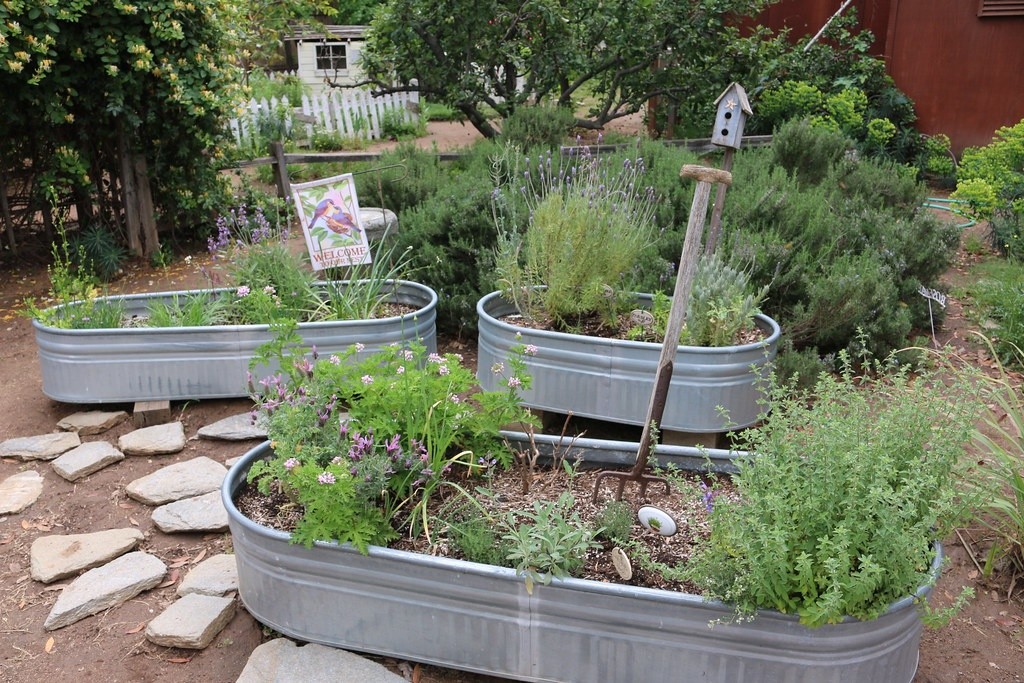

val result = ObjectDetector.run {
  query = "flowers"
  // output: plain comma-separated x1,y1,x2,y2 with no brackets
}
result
4,197,426,327
232,313,1011,635
488,131,786,346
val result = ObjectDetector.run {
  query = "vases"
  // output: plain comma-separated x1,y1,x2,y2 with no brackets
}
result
219,432,945,683
473,284,780,434
31,278,437,405
